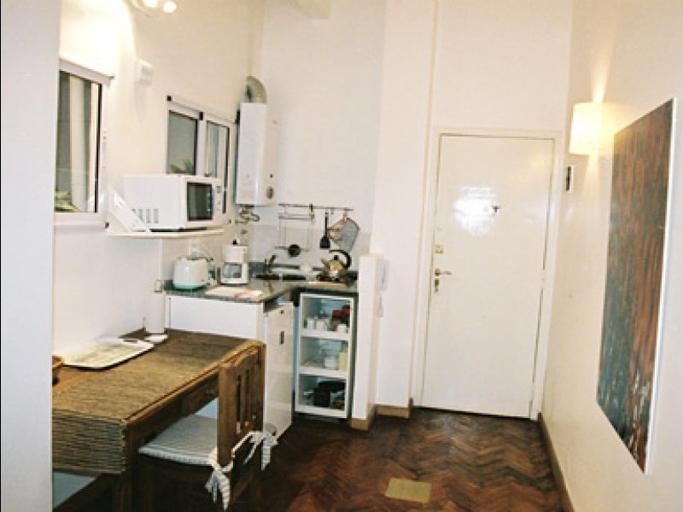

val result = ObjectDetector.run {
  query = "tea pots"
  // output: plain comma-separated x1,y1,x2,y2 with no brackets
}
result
320,248,351,277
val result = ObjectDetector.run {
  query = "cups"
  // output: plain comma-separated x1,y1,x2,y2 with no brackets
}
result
314,388,330,406
316,320,328,331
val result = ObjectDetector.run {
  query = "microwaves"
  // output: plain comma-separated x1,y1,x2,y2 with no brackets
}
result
124,174,223,231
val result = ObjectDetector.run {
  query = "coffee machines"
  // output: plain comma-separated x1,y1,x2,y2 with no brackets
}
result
220,244,248,284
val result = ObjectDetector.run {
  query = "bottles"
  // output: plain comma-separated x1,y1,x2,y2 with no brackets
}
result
208,257,217,285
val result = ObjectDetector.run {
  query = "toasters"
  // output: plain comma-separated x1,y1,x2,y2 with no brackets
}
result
174,255,208,291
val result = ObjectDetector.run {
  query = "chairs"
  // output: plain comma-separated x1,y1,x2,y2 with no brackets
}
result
134,340,262,512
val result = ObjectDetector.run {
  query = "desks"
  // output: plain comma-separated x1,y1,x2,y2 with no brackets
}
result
51,327,264,512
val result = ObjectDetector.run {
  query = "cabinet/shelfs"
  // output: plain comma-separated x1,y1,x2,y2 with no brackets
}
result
295,292,356,423
